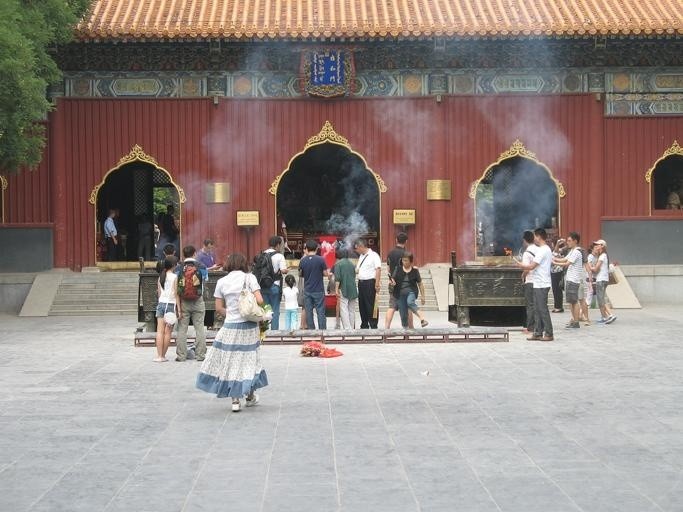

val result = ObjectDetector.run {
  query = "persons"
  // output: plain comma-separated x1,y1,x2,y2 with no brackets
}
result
196,253,268,411
154,205,221,361
519,227,617,341
105,210,119,261
263,232,429,329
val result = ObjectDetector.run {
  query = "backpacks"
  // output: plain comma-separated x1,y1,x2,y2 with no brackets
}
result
250,250,282,289
175,260,204,301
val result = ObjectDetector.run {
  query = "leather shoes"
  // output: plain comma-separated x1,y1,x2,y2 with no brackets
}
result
526,334,543,341
539,335,554,342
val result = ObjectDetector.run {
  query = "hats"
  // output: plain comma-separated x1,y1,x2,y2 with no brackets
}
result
592,239,606,247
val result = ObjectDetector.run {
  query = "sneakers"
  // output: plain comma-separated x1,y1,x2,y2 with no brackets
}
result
551,308,590,328
605,315,618,325
420,318,428,328
244,393,259,407
596,318,607,323
151,355,204,366
230,400,241,412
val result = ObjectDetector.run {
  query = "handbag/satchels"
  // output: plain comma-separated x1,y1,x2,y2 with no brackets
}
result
608,270,620,284
236,273,266,322
163,312,178,326
